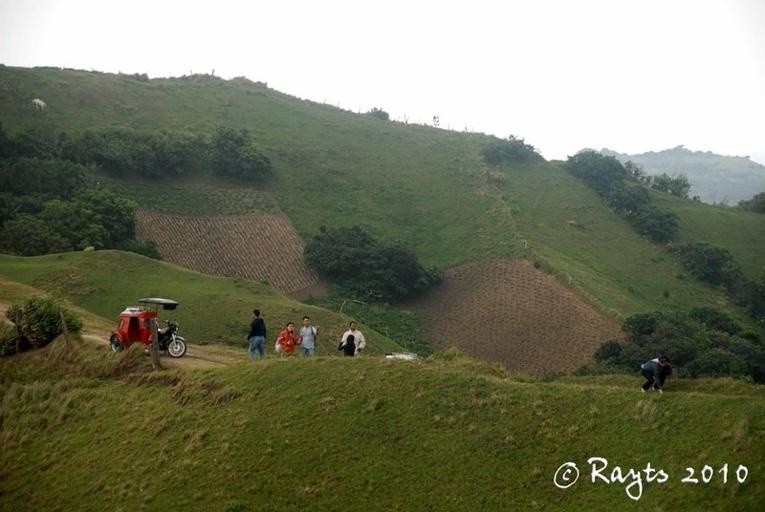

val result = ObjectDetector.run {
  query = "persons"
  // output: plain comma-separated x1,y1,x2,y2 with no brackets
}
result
642,355,672,390
246,308,268,360
300,315,320,356
338,320,366,357
638,357,669,395
278,321,302,358
338,333,356,357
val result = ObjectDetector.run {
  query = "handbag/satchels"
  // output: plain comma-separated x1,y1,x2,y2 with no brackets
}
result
274,337,284,353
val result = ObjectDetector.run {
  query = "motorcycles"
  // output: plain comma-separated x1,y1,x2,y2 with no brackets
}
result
110,297,186,358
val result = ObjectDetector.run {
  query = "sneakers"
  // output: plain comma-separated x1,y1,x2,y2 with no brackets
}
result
640,385,656,393
